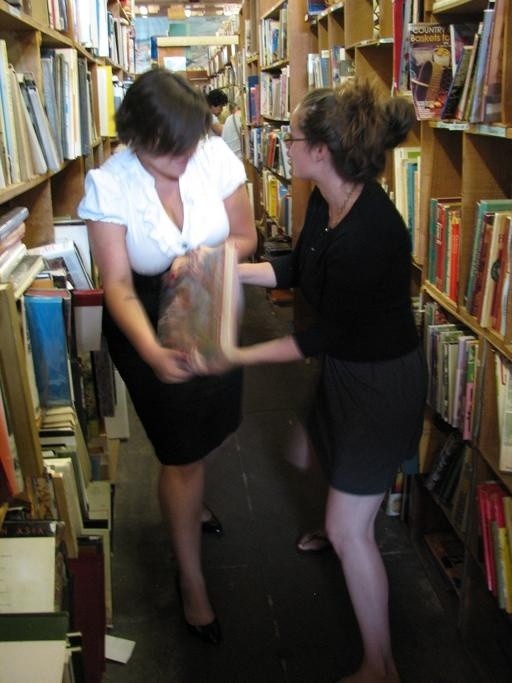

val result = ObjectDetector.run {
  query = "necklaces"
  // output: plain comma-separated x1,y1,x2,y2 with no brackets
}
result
329,178,360,225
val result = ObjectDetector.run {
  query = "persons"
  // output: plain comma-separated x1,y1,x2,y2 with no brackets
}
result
233,78,429,682
77,66,257,650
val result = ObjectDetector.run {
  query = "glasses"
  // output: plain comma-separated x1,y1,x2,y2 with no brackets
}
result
283,132,307,146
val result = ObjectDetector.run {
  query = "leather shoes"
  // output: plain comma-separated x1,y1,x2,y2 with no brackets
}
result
199,502,223,537
173,575,220,645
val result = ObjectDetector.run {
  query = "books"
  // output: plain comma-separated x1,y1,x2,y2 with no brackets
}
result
157,239,235,370
374,1,512,611
1,0,118,682
118,19,138,104
206,0,356,241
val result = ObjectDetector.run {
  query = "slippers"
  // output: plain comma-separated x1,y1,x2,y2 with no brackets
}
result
298,530,330,555
